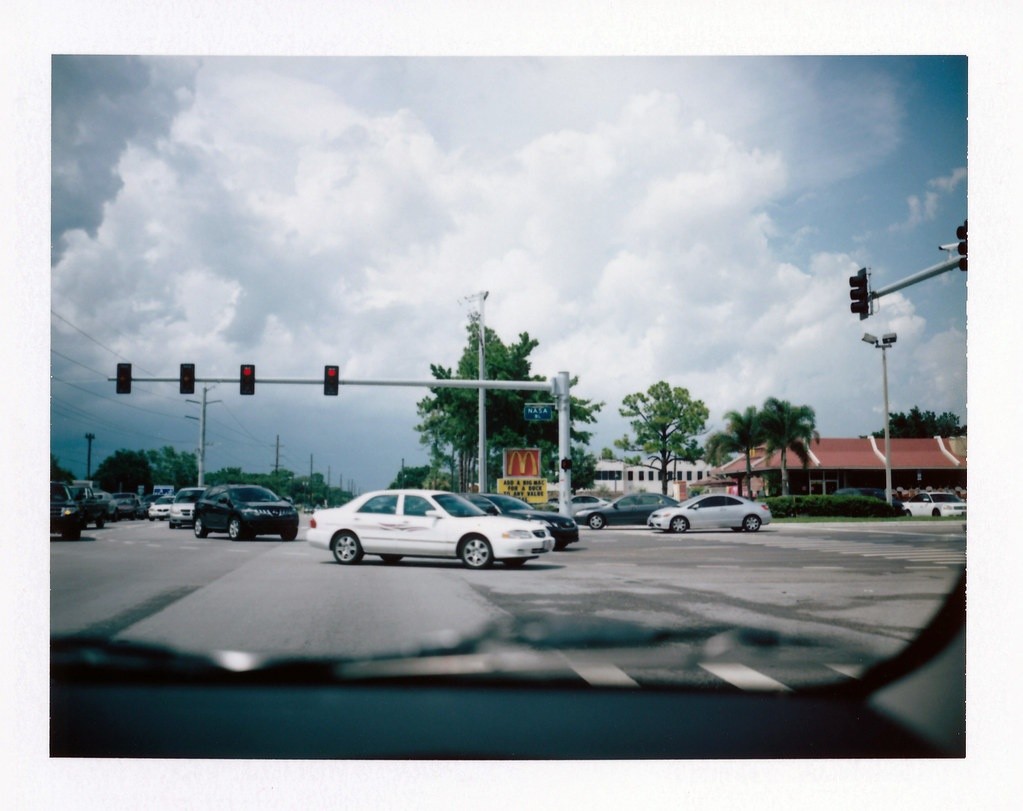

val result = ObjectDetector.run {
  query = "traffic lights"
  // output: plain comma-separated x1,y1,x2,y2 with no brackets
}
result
562,459,574,470
180,364,195,394
956,218,968,271
323,365,339,395
849,268,870,320
116,363,132,394
240,364,255,395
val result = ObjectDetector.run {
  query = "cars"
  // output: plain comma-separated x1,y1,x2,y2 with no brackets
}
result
646,493,774,535
170,487,207,528
148,494,175,521
552,496,611,512
113,494,163,520
418,494,579,551
834,488,904,512
903,491,967,517
575,493,680,530
93,492,120,522
307,489,555,569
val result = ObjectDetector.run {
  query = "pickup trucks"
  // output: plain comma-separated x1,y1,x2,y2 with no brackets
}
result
68,484,109,529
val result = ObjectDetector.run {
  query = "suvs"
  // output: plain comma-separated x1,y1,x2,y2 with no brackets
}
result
50,481,83,539
193,482,299,541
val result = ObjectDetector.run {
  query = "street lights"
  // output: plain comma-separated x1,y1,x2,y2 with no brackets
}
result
862,332,897,506
